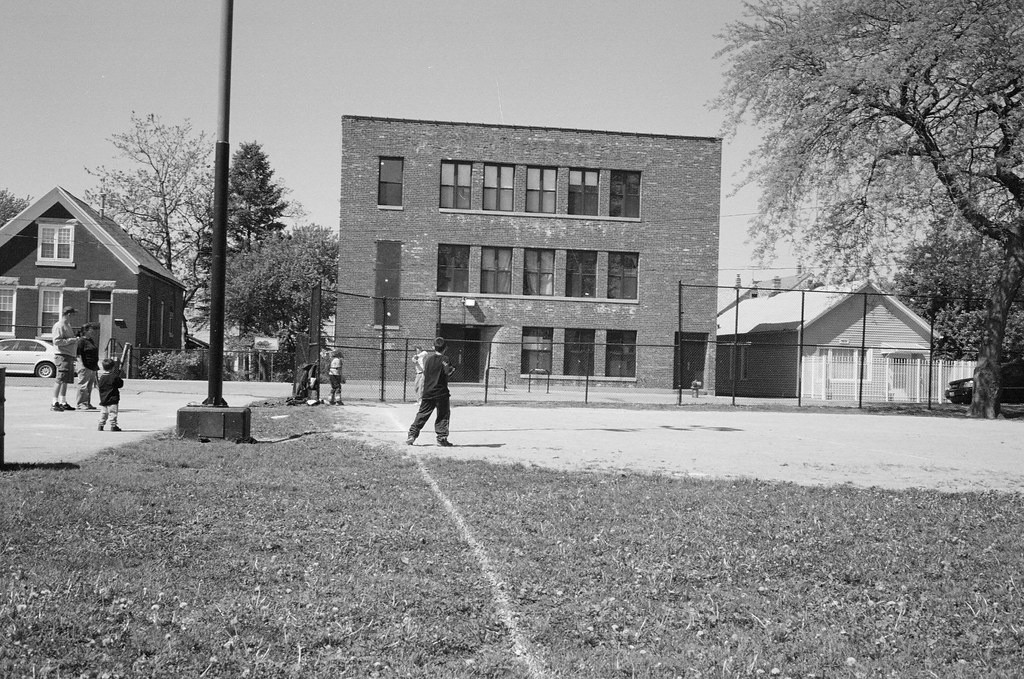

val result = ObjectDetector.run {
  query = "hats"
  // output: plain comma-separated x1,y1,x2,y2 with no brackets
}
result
63,307,78,314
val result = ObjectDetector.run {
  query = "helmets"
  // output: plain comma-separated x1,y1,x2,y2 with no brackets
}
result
332,349,344,357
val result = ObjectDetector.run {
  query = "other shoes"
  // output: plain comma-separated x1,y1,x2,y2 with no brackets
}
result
438,441,453,447
112,426,121,431
414,402,419,406
330,401,344,406
405,437,416,444
98,427,104,431
77,404,97,410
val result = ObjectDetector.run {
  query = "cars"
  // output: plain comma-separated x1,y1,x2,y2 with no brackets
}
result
945,360,1024,404
0,339,62,378
73,356,85,378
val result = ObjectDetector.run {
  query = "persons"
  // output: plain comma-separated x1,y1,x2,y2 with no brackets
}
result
329,349,346,405
406,337,453,446
98,359,124,431
51,306,100,411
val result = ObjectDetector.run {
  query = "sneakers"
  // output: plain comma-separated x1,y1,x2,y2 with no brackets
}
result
50,402,75,411
